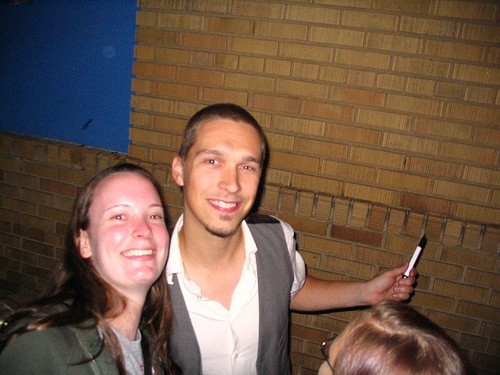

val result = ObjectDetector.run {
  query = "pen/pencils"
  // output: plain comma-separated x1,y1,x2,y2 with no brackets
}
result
402,234,427,279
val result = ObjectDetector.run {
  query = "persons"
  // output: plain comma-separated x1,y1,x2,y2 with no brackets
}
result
317,297,474,375
0,102,418,375
0,154,185,374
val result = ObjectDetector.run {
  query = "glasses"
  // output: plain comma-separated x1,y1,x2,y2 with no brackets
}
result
321,336,339,374
